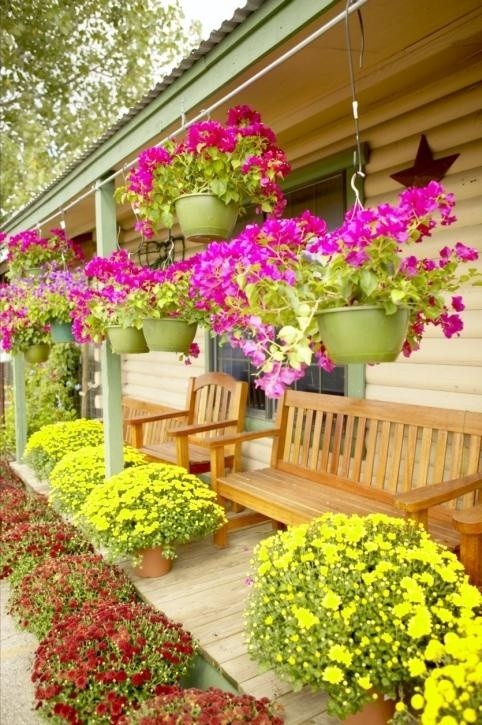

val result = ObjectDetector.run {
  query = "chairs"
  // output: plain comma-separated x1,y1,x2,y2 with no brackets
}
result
124,372,250,515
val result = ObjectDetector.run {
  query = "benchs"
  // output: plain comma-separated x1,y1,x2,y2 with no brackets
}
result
207,389,481,588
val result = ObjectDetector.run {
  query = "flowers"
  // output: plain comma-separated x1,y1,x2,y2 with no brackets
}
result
110,103,288,242
